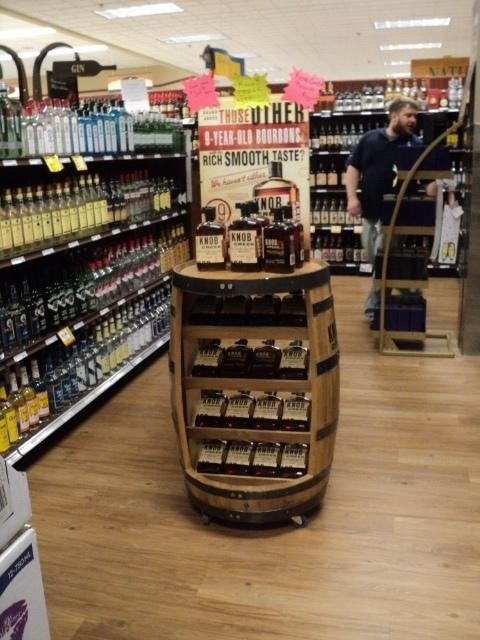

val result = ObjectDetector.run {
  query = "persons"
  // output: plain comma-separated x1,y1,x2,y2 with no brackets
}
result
346,95,438,330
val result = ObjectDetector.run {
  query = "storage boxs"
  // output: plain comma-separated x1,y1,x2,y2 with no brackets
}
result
404,144,450,178
383,191,436,232
377,292,425,350
374,247,430,282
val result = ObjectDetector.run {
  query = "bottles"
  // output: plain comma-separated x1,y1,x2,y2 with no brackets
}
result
247,293,281,325
186,291,221,326
278,441,310,479
250,388,283,430
339,157,349,190
156,221,191,276
309,157,339,191
195,438,229,474
85,299,154,394
252,160,298,222
217,294,249,325
276,340,310,381
0,184,53,260
85,231,158,313
192,205,228,272
457,229,468,267
279,391,313,433
358,169,366,188
278,289,307,328
221,389,256,429
191,338,226,379
151,171,188,215
446,76,466,110
449,158,472,192
1,339,87,456
262,204,306,274
311,231,371,264
0,269,84,351
315,88,334,114
333,87,361,113
53,172,112,248
223,438,257,477
143,90,198,118
218,338,253,378
246,338,282,379
0,79,23,161
146,281,174,339
248,438,285,478
401,235,432,268
365,119,388,130
384,78,430,112
194,388,228,428
77,96,135,157
310,119,365,153
133,111,186,155
361,82,385,112
457,192,468,229
446,115,473,150
311,196,363,228
225,199,270,273
99,167,152,226
19,95,81,159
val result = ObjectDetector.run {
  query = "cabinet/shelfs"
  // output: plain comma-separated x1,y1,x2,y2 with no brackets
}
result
0,97,191,466
170,254,341,530
221,79,465,274
